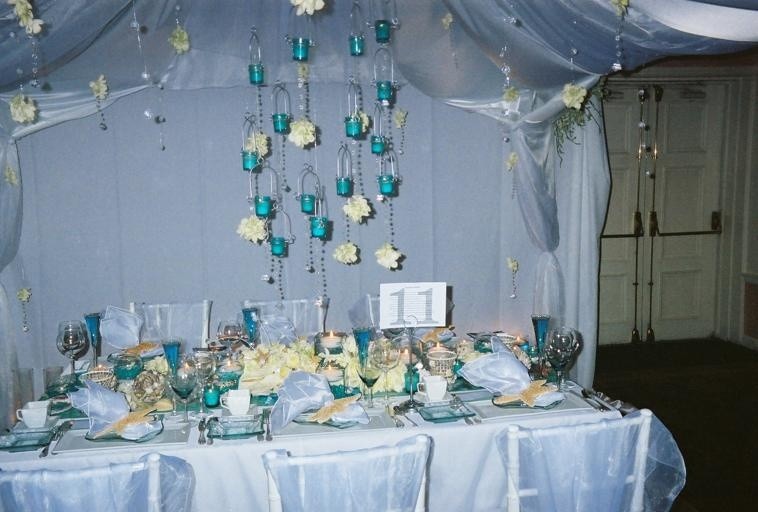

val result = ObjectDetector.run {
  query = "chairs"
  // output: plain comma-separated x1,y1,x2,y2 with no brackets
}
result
243,295,328,345
129,299,212,349
506,408,653,511
0,452,162,511
261,434,434,511
367,294,381,334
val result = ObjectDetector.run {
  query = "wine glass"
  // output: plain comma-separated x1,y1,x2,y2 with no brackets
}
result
54,307,579,428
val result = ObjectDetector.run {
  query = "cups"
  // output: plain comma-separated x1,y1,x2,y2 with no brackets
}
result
13,398,51,429
238,17,397,259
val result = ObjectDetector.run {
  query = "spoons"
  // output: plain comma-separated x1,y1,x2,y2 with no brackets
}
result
582,388,611,416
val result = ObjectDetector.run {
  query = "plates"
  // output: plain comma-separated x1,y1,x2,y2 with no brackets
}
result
87,423,163,443
0,431,53,453
208,421,265,438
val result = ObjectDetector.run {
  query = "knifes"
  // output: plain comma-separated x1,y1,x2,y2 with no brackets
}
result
39,419,76,457
196,416,206,447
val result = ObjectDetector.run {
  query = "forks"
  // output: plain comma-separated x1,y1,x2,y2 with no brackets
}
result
262,406,275,441
